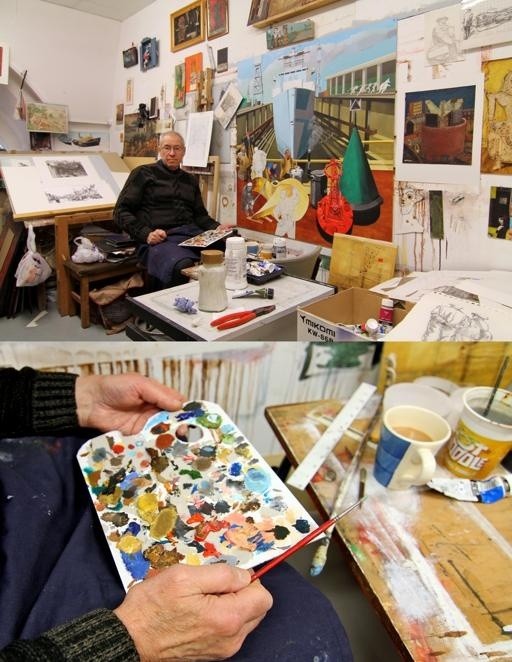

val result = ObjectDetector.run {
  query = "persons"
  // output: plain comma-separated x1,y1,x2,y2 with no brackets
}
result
175,13,198,43
114,132,236,287
0,368,352,660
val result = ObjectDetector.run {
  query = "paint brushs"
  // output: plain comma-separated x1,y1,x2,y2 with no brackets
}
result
250,493,371,584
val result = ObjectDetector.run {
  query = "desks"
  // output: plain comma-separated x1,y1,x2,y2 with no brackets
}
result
120,270,336,341
261,402,512,659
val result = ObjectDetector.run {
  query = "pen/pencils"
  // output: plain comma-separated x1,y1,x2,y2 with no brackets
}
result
359,469,366,509
484,356,509,416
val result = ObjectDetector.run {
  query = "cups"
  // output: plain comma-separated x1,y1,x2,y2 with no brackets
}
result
374,377,511,492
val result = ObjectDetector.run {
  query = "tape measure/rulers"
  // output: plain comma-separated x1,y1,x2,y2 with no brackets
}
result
287,382,377,491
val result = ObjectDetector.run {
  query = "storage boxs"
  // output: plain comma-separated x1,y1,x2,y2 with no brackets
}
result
295,285,416,341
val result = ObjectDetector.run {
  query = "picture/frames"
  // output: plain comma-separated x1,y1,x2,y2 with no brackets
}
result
169,0,229,54
27,103,70,134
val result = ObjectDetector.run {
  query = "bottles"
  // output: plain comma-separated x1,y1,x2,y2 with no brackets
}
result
259,238,287,260
363,298,394,338
198,234,248,310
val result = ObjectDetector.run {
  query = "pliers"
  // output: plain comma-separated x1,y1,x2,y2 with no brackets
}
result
210,304,276,330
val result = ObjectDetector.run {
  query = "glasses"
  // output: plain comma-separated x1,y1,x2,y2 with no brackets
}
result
158,145,185,151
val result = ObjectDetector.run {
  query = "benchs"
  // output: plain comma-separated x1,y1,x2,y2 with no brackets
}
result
53,211,138,326
